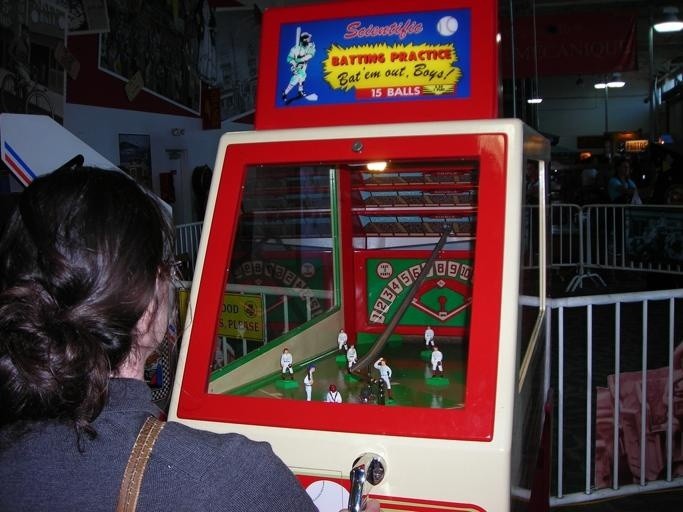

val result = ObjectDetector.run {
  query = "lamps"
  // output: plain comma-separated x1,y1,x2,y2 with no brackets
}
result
652,1,682,34
592,71,626,91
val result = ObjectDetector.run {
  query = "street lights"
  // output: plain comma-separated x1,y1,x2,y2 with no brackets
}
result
526,94,543,128
593,73,626,136
649,0,683,91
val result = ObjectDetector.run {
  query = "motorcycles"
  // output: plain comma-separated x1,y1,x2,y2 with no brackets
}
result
549,168,568,223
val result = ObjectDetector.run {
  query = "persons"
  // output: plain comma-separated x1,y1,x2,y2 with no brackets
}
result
608,160,643,206
524,160,539,205
303,365,315,401
346,345,358,372
0,170,386,512
337,329,348,354
326,385,342,403
424,326,435,350
430,346,443,378
279,32,315,105
358,375,374,403
280,348,293,380
0,0,200,113
374,378,386,405
373,357,393,402
